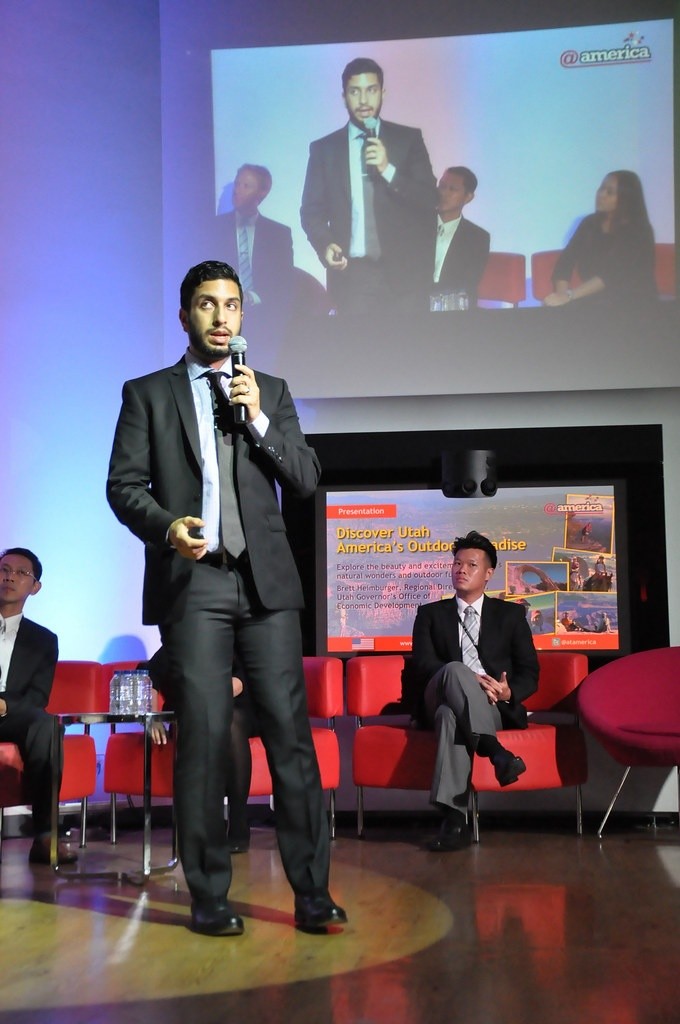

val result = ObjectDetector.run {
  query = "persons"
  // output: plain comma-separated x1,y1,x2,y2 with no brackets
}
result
105,261,348,935
595,612,613,633
0,548,78,864
570,557,580,582
543,170,657,307
562,611,572,624
136,644,259,853
410,530,540,851
430,167,490,308
581,522,592,543
515,599,554,633
595,556,606,573
299,57,439,315
215,164,294,306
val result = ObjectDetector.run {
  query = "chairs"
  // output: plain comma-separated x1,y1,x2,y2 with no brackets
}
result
292,266,330,315
578,645,680,841
347,649,586,844
104,657,342,846
477,253,526,308
531,250,582,301
0,659,101,849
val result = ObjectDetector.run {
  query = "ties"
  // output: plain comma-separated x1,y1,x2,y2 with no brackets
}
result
362,131,381,264
238,219,252,295
208,372,247,559
0,620,6,635
433,226,447,282
461,606,483,675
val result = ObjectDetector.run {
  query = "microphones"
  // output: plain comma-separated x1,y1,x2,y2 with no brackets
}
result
364,117,378,175
228,335,249,424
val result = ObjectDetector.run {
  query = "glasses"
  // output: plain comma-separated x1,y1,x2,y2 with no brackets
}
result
0,566,37,581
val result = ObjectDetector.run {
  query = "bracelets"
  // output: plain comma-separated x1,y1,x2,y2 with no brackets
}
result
566,290,573,301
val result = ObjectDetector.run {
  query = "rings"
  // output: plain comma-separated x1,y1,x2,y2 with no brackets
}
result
246,387,250,393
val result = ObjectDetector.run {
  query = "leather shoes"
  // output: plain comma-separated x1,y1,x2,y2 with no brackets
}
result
495,753,526,786
430,820,472,851
294,892,347,926
30,839,78,864
190,900,243,936
227,836,250,852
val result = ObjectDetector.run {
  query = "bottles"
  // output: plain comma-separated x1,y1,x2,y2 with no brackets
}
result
109,669,152,715
429,288,468,312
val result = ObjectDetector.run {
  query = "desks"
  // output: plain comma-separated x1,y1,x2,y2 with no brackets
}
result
54,709,180,890
